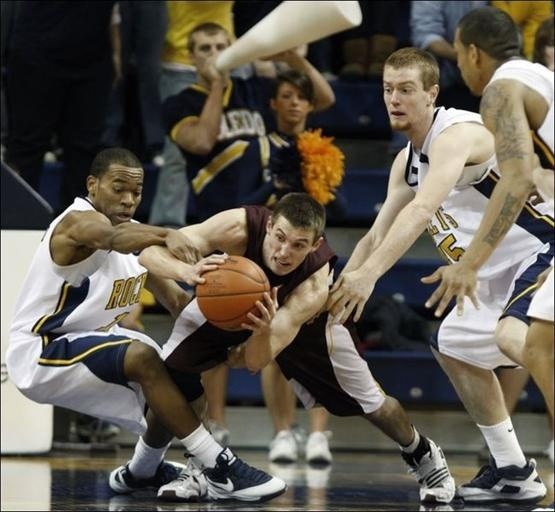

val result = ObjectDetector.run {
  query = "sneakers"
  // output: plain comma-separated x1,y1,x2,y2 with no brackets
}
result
108,433,287,502
306,431,334,468
456,454,548,505
269,428,299,462
400,435,456,505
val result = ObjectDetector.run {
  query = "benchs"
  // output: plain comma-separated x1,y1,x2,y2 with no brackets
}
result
224,254,554,417
43,2,411,229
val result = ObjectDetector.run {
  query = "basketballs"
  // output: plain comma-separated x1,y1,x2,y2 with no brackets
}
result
196,256,271,331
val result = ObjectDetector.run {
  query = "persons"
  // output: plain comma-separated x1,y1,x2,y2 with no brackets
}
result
342,0,555,115
0,146,289,505
108,190,458,506
419,7,555,433
325,46,555,506
236,67,334,467
161,22,339,464
0,0,237,225
